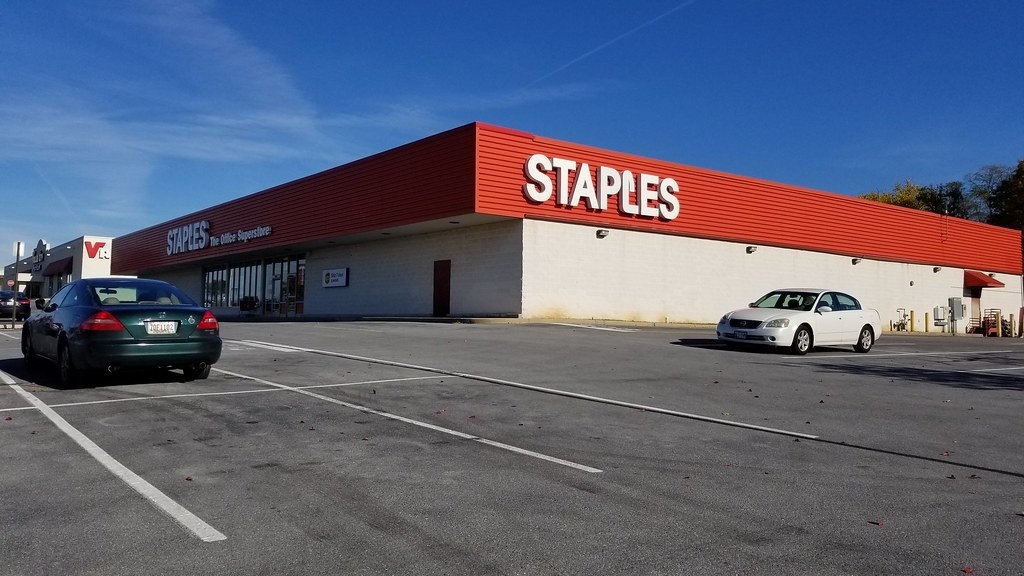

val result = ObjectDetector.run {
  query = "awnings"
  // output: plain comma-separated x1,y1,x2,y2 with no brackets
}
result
965,270,1006,288
42,256,72,276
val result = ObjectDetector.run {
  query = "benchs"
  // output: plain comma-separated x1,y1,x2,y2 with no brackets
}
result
238,300,257,318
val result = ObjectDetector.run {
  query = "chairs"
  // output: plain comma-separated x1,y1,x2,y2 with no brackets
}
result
157,297,172,304
788,299,799,308
102,297,120,304
818,301,829,307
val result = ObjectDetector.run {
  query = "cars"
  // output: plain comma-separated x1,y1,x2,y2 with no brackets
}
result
716,287,882,356
21,277,222,387
0,290,32,323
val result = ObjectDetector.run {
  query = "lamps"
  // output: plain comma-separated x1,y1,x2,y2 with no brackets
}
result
852,258,861,265
746,246,757,254
932,267,941,273
596,229,609,238
